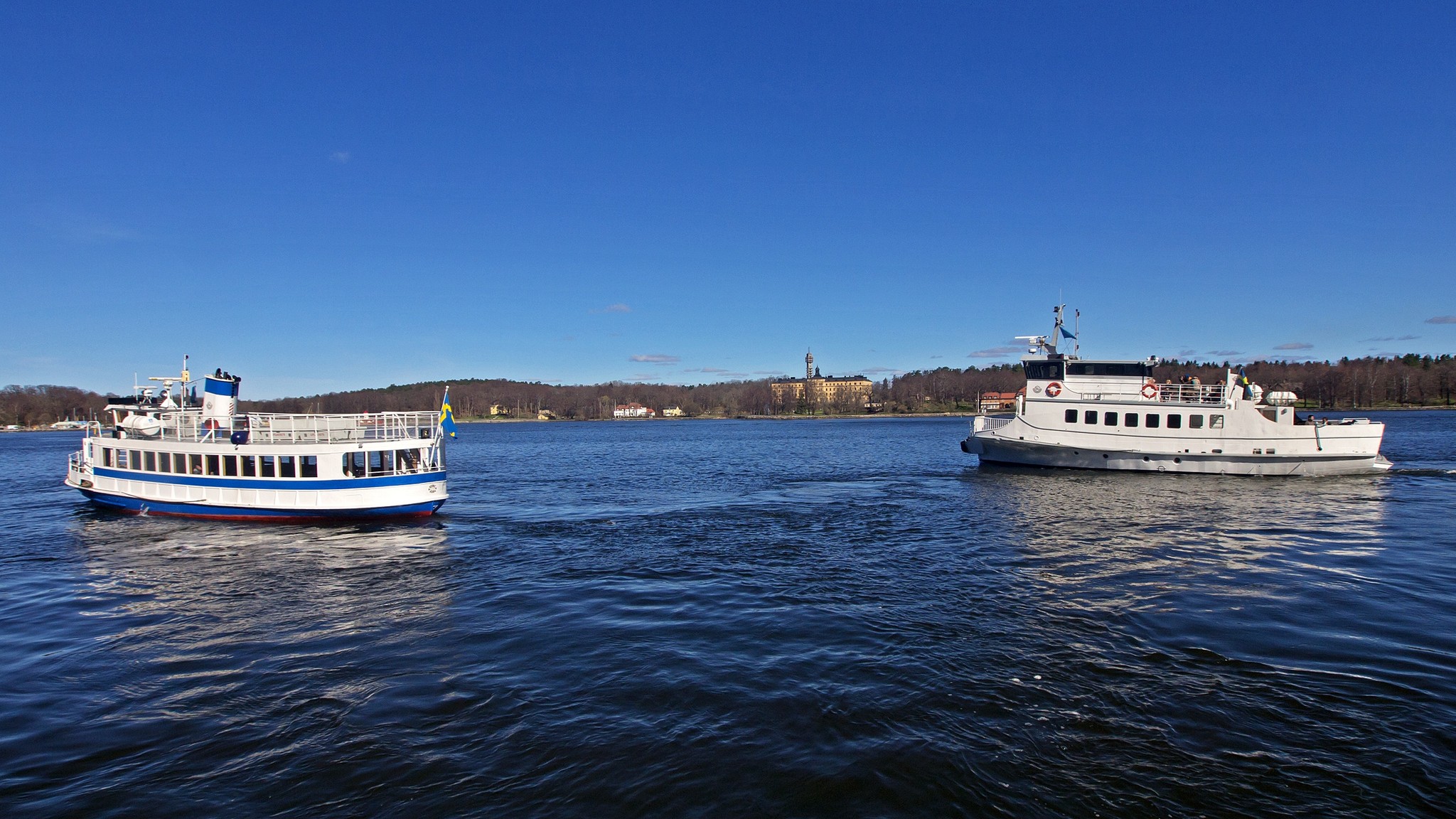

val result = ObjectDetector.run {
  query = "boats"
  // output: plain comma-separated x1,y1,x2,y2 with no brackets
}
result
63,353,450,521
959,289,1395,476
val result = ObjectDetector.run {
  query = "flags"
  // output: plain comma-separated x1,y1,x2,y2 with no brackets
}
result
1237,369,1252,397
1060,327,1077,340
440,392,458,439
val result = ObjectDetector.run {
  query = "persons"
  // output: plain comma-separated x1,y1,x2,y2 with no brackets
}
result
1179,374,1200,403
1213,380,1226,404
1162,380,1173,402
1323,417,1329,425
1305,415,1314,425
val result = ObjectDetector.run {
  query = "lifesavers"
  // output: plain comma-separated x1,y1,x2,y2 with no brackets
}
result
1142,384,1158,399
1047,382,1062,397
